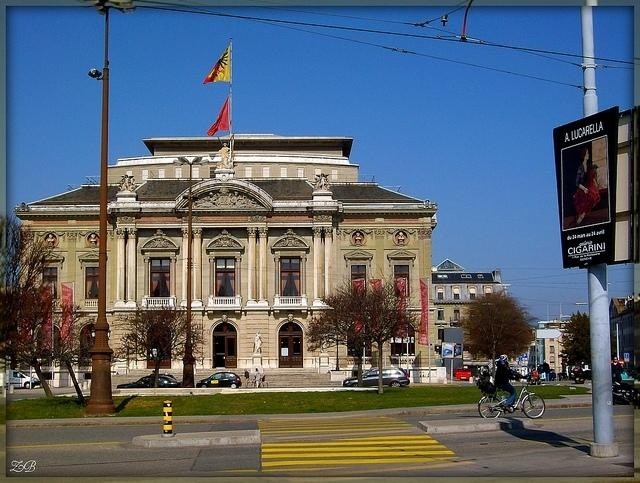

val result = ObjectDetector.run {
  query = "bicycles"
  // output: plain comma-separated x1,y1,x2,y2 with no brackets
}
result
477,372,546,419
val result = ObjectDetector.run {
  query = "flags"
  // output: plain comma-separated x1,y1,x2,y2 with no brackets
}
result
36,281,57,355
201,36,233,86
418,277,431,346
205,93,232,136
369,278,385,336
15,284,34,358
61,280,76,351
350,278,367,334
394,277,410,338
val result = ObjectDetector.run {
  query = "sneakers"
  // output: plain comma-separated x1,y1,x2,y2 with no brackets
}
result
505,406,512,413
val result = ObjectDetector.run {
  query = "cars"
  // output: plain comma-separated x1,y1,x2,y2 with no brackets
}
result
8,370,41,389
116,374,178,388
195,371,242,389
343,366,410,388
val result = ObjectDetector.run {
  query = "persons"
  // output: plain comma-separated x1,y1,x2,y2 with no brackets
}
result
244,368,250,387
531,367,540,383
253,368,261,388
218,142,231,167
496,354,530,412
120,174,132,191
611,356,624,386
252,330,264,355
570,143,601,229
541,359,551,384
315,172,329,192
258,372,265,387
